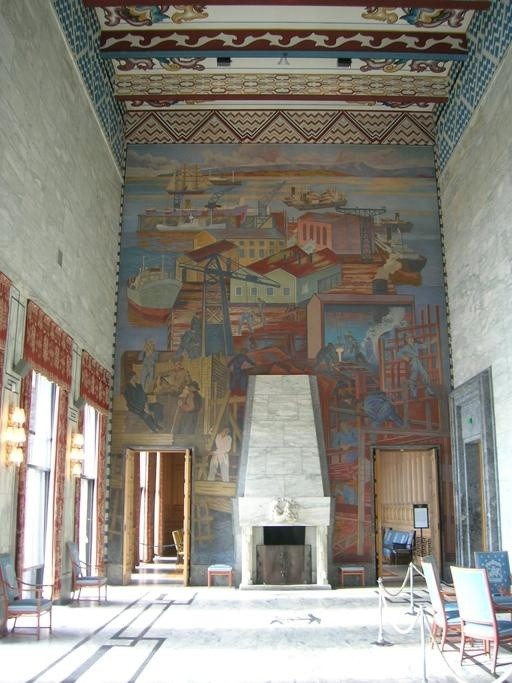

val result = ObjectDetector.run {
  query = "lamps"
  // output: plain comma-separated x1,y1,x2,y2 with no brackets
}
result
67,427,86,479
4,404,26,467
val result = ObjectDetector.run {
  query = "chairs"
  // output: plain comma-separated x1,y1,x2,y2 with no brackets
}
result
384,525,416,563
420,561,486,658
0,552,56,639
444,563,512,674
172,531,184,565
66,537,112,604
474,549,512,613
422,554,468,640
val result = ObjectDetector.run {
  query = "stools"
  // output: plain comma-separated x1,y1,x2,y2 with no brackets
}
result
339,565,367,586
207,561,233,589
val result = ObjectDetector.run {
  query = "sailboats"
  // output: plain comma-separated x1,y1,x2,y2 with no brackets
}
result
162,158,215,197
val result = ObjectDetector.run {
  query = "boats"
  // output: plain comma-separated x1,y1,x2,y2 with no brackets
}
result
283,182,350,212
379,233,430,273
206,168,249,186
124,251,183,319
153,216,228,232
380,212,416,233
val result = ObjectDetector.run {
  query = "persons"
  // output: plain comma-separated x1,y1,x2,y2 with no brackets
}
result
121,339,232,484
315,330,435,463
